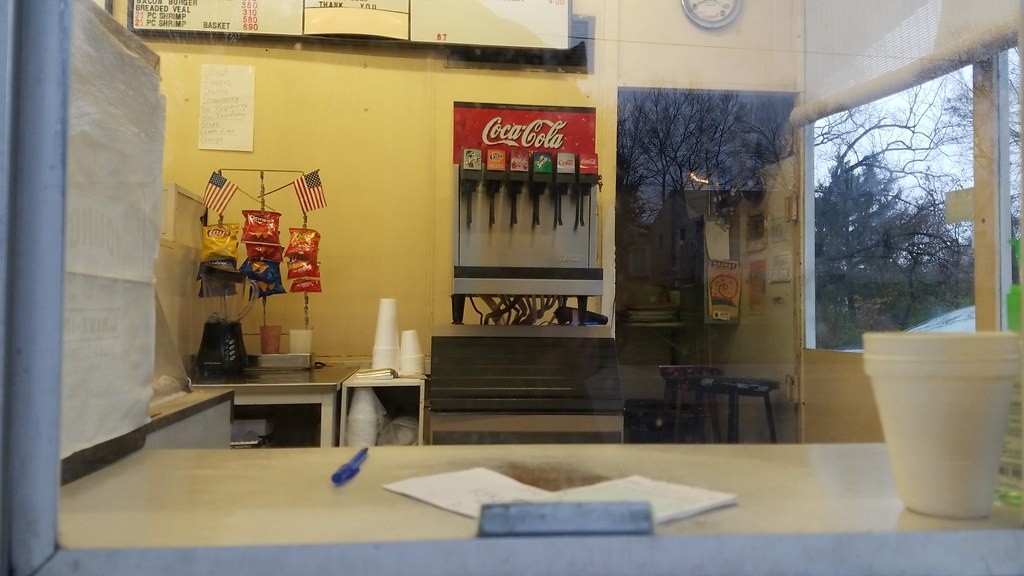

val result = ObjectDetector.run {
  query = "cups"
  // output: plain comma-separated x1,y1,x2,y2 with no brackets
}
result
371,295,427,376
346,385,377,449
860,327,1022,521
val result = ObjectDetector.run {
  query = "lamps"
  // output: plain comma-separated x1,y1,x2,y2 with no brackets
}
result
681,0,742,31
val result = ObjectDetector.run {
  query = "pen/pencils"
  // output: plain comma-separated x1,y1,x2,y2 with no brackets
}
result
330,447,368,486
